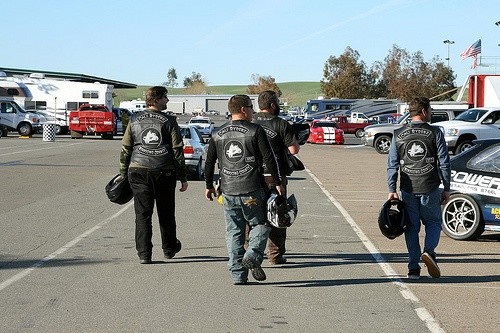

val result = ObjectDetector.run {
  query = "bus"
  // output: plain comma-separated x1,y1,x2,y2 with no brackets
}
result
306,99,399,114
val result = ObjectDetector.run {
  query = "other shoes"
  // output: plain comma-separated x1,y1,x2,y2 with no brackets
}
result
163,238,181,259
421,251,441,280
242,256,266,281
139,255,152,264
269,256,286,265
406,268,421,279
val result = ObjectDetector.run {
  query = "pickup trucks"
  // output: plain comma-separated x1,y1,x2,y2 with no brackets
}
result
329,117,373,138
204,110,220,115
331,112,370,124
69,104,115,140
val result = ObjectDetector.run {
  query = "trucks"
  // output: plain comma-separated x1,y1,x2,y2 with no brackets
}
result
0,73,114,136
0,72,41,137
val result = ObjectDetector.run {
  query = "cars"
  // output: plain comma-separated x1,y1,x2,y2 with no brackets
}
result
178,124,209,180
433,108,500,155
306,120,345,145
363,108,468,155
440,138,500,241
278,112,304,122
188,117,215,137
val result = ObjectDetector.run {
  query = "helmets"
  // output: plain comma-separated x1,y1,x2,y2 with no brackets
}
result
378,198,407,240
105,174,134,205
285,147,294,174
266,192,298,228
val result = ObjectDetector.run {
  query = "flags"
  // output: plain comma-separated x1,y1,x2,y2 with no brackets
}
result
461,40,481,58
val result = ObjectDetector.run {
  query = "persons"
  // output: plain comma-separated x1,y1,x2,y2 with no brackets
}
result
120,86,189,264
205,95,286,285
243,91,301,263
387,96,451,279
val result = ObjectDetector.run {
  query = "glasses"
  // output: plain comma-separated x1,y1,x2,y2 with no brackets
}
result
163,95,167,98
245,105,253,109
431,108,433,111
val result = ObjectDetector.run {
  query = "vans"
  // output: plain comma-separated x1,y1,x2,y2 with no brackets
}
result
113,108,133,135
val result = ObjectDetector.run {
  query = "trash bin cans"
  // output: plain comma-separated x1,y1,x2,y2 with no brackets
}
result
43,123,55,142
